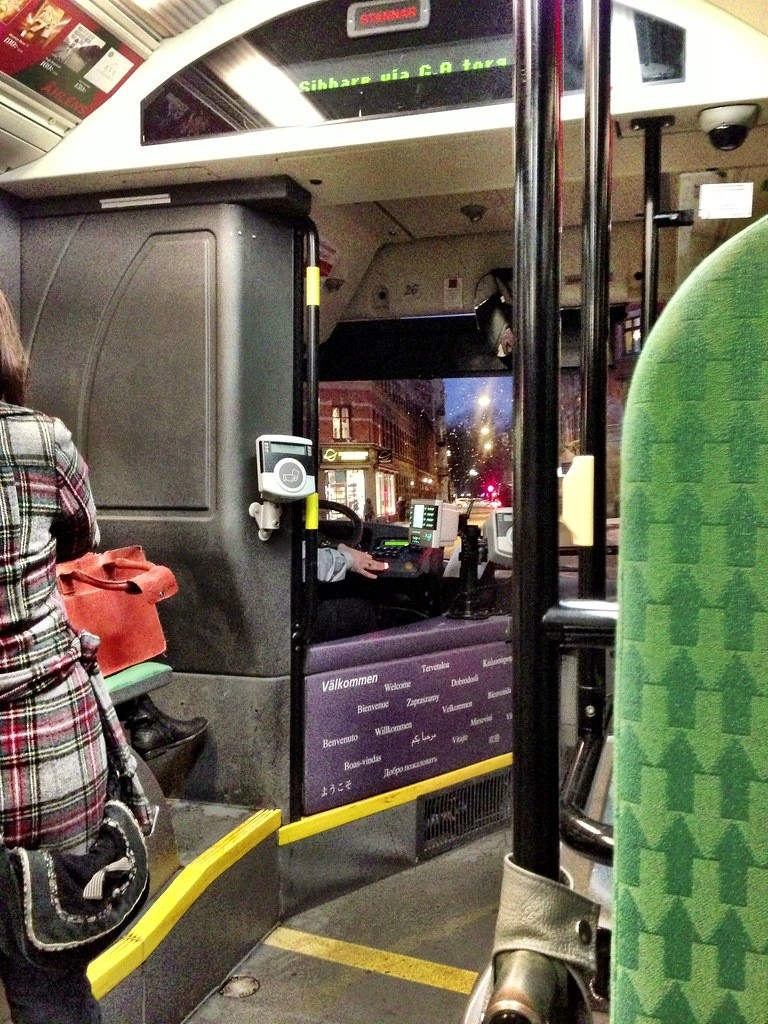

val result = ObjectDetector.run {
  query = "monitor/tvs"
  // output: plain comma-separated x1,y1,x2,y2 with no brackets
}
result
409,500,460,541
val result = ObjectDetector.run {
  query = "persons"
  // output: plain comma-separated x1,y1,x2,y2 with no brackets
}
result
315,543,389,641
364,498,376,522
394,496,406,522
0,290,162,1024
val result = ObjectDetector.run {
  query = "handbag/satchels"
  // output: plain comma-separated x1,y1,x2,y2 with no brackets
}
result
54,545,179,678
0,799,150,968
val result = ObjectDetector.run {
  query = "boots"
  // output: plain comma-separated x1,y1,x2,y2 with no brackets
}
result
125,693,210,761
